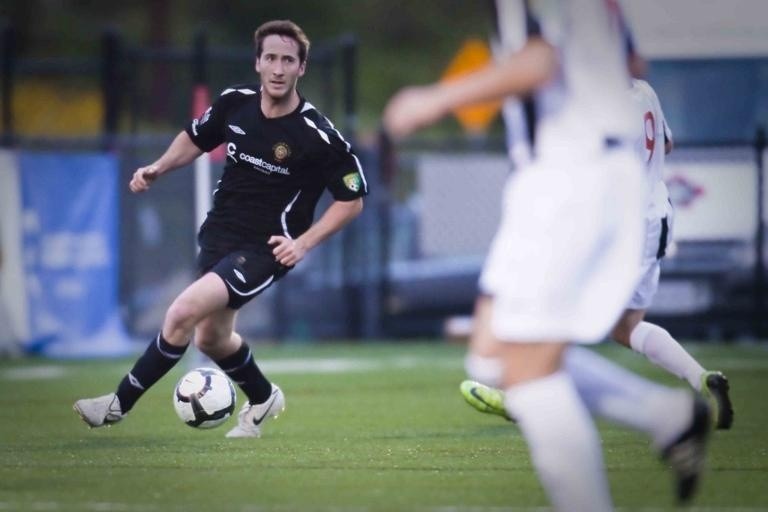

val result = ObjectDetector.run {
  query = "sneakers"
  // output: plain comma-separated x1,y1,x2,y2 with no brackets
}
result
459,378,520,425
226,383,286,439
657,398,715,503
73,392,129,429
699,370,734,432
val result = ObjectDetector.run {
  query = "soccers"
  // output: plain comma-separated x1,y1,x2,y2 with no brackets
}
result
173,367,237,429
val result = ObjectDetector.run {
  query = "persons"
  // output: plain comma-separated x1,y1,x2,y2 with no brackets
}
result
457,26,735,431
70,19,370,439
379,2,712,511
